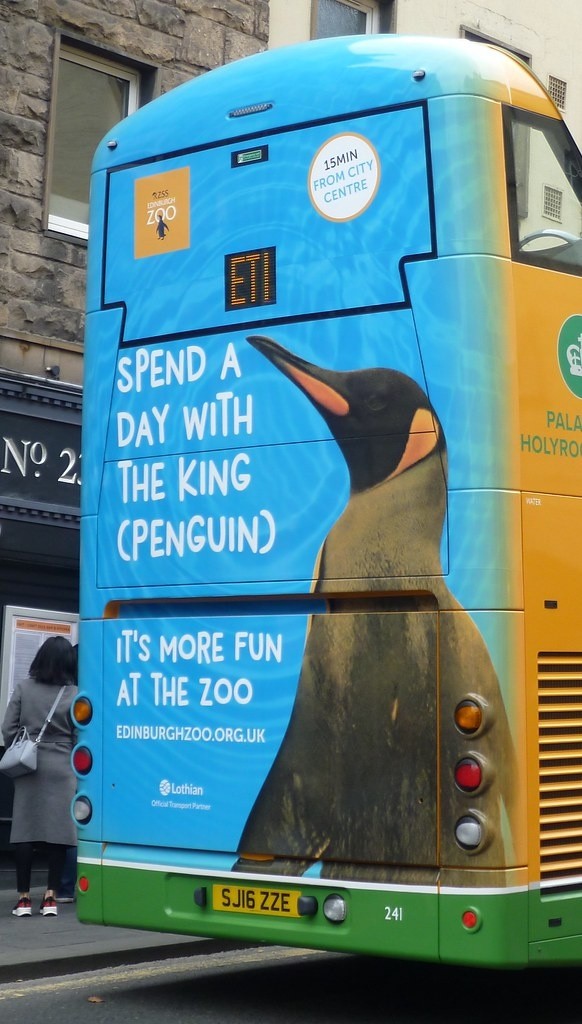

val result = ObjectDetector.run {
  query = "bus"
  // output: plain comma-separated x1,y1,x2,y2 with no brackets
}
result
67,35,581,970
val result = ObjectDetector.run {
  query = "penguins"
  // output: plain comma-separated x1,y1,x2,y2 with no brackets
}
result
231,335,530,894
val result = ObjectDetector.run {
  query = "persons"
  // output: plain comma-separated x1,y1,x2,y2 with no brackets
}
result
1,636,79,919
50,643,78,902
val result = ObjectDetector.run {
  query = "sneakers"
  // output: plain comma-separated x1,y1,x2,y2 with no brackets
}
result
12,896,32,917
40,893,58,916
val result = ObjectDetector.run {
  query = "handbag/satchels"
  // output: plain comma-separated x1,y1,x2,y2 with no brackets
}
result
0,725,39,779
